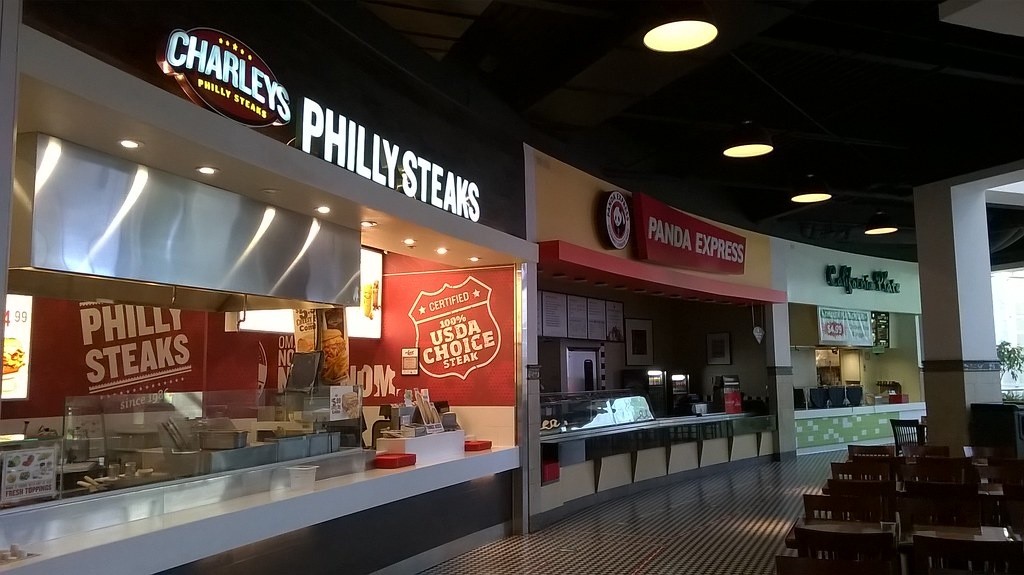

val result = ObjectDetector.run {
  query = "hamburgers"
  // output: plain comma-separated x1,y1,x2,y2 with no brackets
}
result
322,329,346,355
3,338,26,374
342,392,357,410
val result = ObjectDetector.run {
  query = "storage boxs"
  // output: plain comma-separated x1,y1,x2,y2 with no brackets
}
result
464,440,492,452
376,451,417,468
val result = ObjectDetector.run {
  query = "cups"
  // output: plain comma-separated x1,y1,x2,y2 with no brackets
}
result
287,464,320,492
373,280,379,307
363,284,372,317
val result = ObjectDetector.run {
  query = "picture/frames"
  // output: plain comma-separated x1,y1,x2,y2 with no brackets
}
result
624,318,655,366
401,347,419,377
705,332,732,365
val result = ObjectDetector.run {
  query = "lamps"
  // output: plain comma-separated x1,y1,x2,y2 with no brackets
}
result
864,210,899,235
790,172,831,203
639,0,720,53
721,121,774,159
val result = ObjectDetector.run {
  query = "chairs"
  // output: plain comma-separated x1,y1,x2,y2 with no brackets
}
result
772,419,1024,575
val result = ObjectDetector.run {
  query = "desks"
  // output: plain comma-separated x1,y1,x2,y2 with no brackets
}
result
847,455,905,487
927,481,1006,525
785,517,893,555
906,457,970,474
904,525,1016,545
821,478,901,495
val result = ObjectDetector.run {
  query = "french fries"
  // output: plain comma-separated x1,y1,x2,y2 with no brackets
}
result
323,339,349,380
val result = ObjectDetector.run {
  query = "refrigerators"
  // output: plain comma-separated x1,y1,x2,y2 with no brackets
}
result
622,369,701,418
